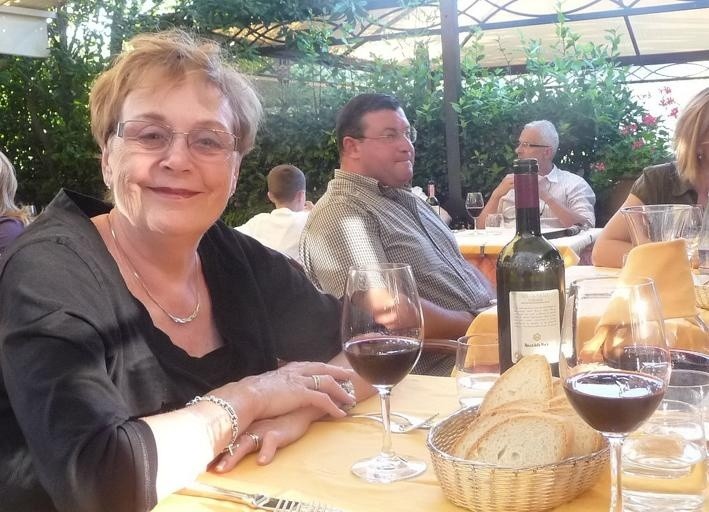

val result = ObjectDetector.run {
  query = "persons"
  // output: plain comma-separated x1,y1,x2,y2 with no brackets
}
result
0,150,29,261
589,87,709,273
231,164,317,268
0,25,394,511
297,96,497,376
472,119,597,234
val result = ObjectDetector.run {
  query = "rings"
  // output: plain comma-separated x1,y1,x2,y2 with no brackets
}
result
311,372,324,392
242,428,262,451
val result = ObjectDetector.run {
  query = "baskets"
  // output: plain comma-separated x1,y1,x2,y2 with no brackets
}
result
426,403,611,512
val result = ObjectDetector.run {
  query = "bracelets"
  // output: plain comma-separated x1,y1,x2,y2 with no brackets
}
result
465,307,481,316
546,197,555,207
183,389,241,456
332,377,357,414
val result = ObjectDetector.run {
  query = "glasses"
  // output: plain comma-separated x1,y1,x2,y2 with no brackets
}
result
517,141,551,148
381,125,417,144
115,119,240,163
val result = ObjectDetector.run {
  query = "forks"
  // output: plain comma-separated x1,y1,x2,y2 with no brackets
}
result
317,406,438,436
369,408,442,429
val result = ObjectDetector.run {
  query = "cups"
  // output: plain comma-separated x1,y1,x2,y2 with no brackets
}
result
612,368,709,511
21,202,37,216
484,213,504,236
621,205,704,255
454,336,499,408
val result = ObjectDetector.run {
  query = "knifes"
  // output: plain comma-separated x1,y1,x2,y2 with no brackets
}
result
165,475,342,511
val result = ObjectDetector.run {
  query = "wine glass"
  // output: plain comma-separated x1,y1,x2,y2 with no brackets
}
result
338,258,431,481
465,192,484,238
553,272,672,508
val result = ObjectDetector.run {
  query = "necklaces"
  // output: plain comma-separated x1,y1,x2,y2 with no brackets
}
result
108,207,206,333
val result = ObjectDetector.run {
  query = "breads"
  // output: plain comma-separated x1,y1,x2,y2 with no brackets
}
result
450,354,603,469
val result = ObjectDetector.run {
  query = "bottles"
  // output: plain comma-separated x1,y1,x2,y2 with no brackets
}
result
494,157,567,379
425,179,440,217
